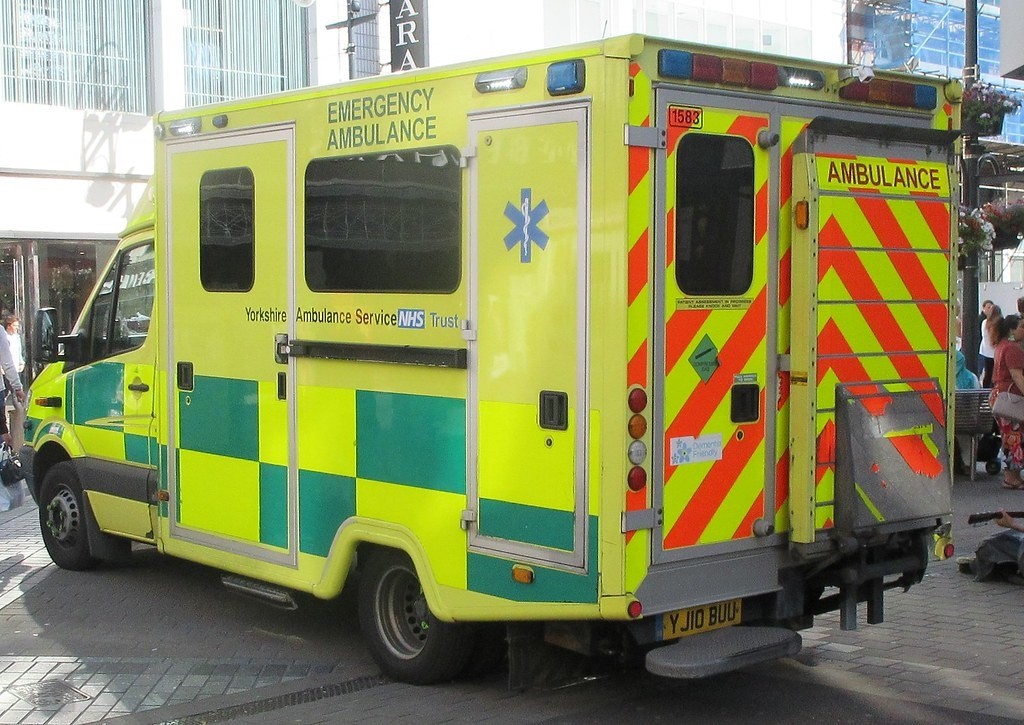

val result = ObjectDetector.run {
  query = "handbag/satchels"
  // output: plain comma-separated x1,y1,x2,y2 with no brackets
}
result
991,381,1024,422
0,441,25,512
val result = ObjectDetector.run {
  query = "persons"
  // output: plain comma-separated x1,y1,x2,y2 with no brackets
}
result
953,295,1024,586
0,310,25,446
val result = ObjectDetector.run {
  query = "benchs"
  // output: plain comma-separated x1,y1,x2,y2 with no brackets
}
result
954,388,994,481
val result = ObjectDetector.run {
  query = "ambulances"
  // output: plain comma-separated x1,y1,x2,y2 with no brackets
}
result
15,32,962,689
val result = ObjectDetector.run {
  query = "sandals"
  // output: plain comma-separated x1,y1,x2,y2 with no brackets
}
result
1002,479,1024,490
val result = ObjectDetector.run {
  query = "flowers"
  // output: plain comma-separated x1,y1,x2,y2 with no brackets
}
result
961,81,1022,128
958,198,1024,257
